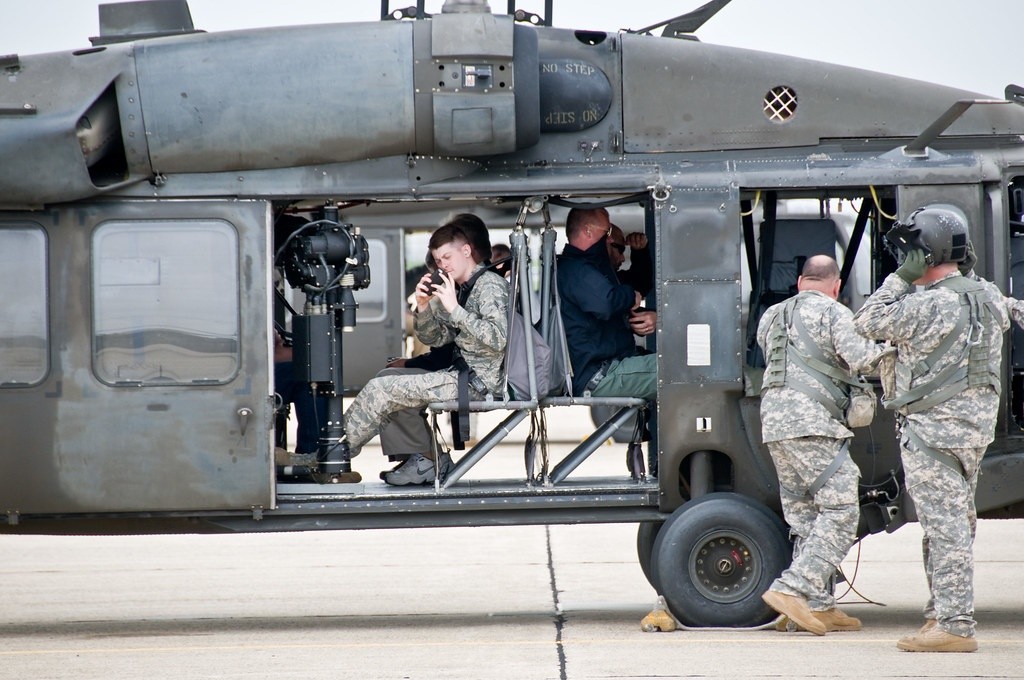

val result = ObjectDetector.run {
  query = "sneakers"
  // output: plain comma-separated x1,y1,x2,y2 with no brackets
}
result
797,608,862,632
762,589,825,636
897,620,978,653
385,448,455,486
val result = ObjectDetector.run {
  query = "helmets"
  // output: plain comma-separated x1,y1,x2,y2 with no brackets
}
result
889,201,969,264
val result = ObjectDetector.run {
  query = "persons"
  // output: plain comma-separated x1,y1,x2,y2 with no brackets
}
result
273,214,514,490
556,203,658,401
852,204,1012,653
608,223,656,302
755,255,893,637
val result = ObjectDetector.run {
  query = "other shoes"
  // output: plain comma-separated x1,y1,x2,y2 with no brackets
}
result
274,447,304,469
380,460,408,484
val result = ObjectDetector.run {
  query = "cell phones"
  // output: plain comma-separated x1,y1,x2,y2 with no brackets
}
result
420,268,448,296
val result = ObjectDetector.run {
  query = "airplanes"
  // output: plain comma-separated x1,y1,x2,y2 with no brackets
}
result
1,0,1024,628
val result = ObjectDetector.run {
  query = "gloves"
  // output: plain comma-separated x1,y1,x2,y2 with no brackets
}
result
895,249,928,286
958,240,977,277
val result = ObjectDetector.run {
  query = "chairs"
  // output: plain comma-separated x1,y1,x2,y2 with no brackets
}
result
540,228,648,487
428,230,536,491
747,216,840,369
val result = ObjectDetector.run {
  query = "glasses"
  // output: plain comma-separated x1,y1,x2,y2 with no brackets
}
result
589,223,612,236
611,242,625,254
